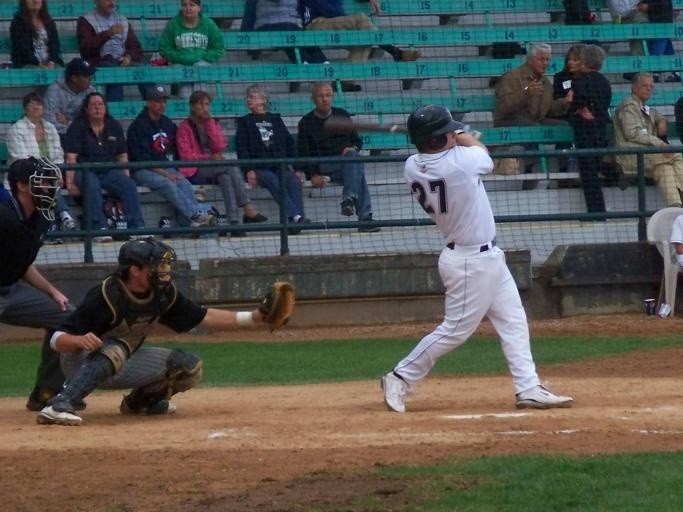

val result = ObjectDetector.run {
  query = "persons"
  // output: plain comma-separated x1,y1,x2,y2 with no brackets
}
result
77,1,159,100
160,2,226,96
0,155,90,412
6,93,77,243
180,91,269,223
127,84,217,227
44,59,100,136
670,213,683,295
377,103,575,414
241,1,420,93
10,1,63,92
299,83,381,232
493,0,682,222
63,94,145,240
35,234,271,426
234,84,312,223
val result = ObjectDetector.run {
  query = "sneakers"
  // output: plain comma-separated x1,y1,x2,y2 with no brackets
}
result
33,403,86,426
25,390,88,412
119,395,178,417
380,370,407,414
287,213,310,226
613,164,629,192
189,211,268,240
357,218,381,232
340,198,354,216
516,384,575,409
43,220,155,243
445,238,500,252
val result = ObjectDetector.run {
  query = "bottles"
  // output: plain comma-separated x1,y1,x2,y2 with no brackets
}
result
149,51,164,65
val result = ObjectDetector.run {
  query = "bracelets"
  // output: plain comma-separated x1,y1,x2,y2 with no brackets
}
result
235,309,254,327
453,128,467,136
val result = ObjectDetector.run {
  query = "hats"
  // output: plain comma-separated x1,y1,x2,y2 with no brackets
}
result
64,56,96,77
144,82,171,100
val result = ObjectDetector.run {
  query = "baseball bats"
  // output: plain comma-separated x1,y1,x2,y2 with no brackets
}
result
326,118,481,140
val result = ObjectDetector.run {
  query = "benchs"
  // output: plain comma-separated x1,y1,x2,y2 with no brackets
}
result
0,0,683,202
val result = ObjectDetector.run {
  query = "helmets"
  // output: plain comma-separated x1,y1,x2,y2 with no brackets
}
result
406,103,467,147
9,153,65,224
115,235,177,295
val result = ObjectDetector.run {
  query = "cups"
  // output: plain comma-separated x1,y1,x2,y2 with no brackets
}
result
643,298,656,316
658,302,671,319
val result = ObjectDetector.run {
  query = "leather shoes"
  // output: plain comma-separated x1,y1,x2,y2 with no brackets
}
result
557,180,580,189
395,48,422,61
521,164,543,191
341,83,363,91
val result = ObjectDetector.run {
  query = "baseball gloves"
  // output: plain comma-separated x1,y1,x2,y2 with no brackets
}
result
258,282,295,332
